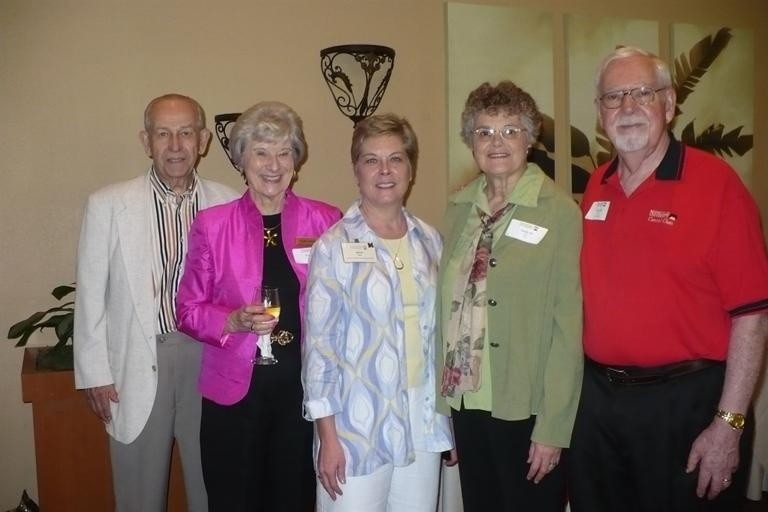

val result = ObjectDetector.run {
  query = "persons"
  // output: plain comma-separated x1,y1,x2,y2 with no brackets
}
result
434,81,583,512
301,114,458,512
74,94,241,512
174,102,344,512
568,46,768,512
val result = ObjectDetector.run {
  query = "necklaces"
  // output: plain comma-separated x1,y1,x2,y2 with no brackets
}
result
382,238,404,270
263,224,283,248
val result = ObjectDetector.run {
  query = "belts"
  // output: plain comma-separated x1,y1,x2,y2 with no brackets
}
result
584,356,722,385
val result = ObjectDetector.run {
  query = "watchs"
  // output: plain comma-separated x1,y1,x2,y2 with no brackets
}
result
715,408,745,435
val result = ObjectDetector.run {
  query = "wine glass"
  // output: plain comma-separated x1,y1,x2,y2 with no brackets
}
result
249,285,281,366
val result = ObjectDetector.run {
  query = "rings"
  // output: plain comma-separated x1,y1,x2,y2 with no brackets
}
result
318,475,323,481
549,462,557,466
251,323,254,332
723,478,734,482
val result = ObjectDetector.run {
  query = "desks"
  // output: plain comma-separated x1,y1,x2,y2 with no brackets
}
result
22,346,187,512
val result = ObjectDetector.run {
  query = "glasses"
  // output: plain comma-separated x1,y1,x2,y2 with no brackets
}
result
470,123,528,139
595,86,668,111
